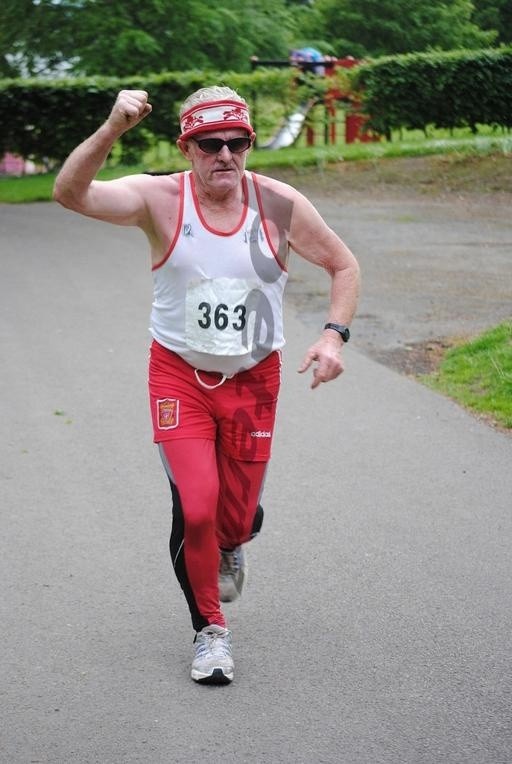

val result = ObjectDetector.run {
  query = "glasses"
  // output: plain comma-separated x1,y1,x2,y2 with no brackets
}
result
190,132,252,155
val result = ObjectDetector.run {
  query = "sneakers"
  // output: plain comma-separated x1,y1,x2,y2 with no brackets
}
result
216,544,248,605
188,623,236,689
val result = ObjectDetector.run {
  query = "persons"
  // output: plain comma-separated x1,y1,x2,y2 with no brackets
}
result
53,84,365,689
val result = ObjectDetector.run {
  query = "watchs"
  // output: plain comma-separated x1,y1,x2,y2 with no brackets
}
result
324,321,351,342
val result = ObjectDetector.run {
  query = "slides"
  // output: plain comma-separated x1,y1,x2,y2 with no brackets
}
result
257,95,317,151
